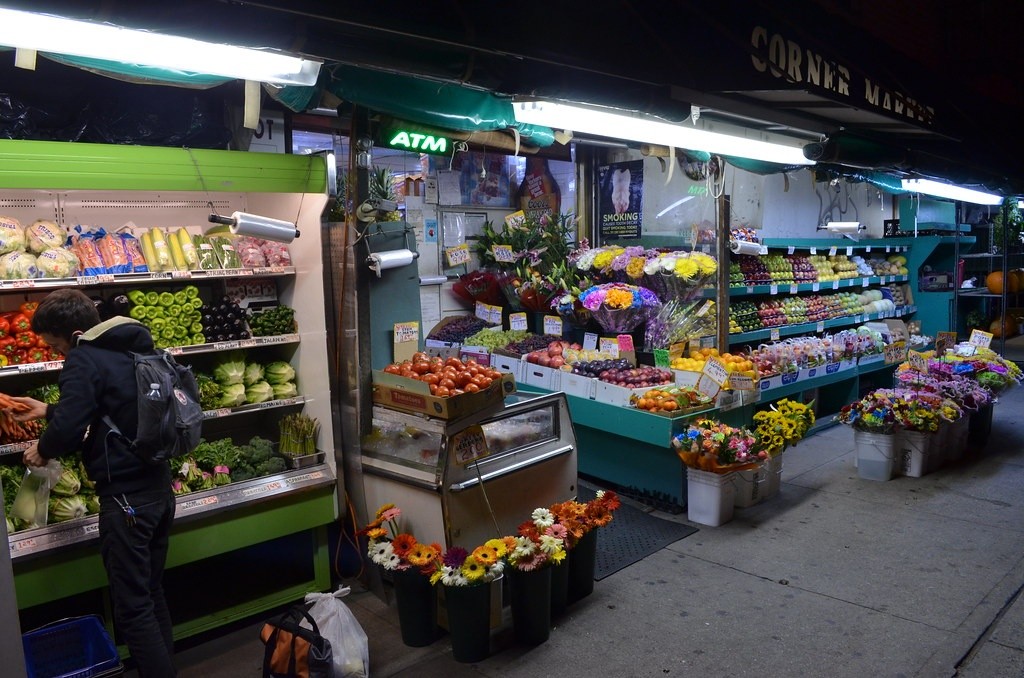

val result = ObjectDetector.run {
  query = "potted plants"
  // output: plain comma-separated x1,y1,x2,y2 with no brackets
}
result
369,161,399,215
992,197,1023,255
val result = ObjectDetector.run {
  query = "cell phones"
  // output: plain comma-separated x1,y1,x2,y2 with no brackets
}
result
70,335,81,349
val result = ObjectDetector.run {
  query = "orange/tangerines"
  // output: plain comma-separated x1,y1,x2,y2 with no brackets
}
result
561,348,613,373
670,346,759,393
636,387,709,414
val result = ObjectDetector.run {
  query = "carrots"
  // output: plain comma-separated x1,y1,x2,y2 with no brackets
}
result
0,392,43,442
70,232,147,275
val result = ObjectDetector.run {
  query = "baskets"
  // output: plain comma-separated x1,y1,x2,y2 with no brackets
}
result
21,613,124,678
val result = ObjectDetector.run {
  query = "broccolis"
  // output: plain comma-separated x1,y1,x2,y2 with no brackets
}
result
230,435,289,483
0,217,80,280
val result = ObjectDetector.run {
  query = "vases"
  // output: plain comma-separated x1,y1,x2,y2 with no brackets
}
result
571,324,597,347
602,330,634,338
545,548,570,625
533,311,553,336
392,572,438,648
508,560,551,646
634,347,656,368
969,402,994,442
569,527,599,599
443,582,491,664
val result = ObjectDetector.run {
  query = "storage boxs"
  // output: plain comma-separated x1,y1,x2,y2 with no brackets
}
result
924,275,950,289
884,219,953,237
522,360,556,389
424,325,502,362
590,378,675,408
760,319,912,391
371,369,506,420
554,369,593,399
490,346,549,383
459,346,489,366
271,441,326,470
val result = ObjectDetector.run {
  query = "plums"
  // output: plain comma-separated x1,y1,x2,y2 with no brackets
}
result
571,357,672,389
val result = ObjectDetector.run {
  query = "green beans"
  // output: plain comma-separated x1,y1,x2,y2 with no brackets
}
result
193,234,243,270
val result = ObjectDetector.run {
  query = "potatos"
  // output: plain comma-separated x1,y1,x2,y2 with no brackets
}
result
890,327,906,343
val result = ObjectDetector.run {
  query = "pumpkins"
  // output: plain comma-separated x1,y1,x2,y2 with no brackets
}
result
987,266,1024,339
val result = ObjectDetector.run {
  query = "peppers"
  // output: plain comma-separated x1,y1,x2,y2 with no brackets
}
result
0,285,298,368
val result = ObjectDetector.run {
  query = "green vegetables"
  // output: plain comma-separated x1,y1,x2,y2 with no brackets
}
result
23,367,226,437
0,436,243,537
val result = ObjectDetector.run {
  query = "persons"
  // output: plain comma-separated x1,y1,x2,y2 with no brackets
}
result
2,288,176,678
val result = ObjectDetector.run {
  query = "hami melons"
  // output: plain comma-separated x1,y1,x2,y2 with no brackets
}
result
885,255,908,275
855,289,894,313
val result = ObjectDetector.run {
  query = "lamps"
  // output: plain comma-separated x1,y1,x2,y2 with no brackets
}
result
900,174,1005,206
510,95,817,166
0,2,325,86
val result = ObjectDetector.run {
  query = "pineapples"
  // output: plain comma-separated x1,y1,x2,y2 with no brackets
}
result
329,167,402,222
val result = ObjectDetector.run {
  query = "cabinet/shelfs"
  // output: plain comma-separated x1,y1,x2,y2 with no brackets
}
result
614,238,936,442
0,264,338,661
952,200,1024,367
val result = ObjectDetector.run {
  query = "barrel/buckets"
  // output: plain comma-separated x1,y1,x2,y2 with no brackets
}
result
687,451,784,527
855,416,971,478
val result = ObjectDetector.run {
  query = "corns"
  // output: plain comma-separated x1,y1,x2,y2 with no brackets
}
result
140,227,197,271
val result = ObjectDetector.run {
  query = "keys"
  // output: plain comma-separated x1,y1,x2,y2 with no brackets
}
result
127,508,136,527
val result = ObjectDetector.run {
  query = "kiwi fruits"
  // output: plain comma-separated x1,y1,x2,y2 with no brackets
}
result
888,283,905,306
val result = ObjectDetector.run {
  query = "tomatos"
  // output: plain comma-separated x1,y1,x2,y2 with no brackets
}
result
384,350,501,398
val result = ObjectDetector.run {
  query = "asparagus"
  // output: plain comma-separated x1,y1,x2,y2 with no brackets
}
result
278,411,320,457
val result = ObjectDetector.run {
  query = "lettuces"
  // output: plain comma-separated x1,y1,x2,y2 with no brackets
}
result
205,348,297,406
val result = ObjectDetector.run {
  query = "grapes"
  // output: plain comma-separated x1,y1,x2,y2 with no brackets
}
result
428,314,563,356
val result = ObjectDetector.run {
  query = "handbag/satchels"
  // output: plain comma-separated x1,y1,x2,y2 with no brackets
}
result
300,588,369,678
259,607,334,678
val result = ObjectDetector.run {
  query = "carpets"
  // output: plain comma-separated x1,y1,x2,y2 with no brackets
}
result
577,483,700,582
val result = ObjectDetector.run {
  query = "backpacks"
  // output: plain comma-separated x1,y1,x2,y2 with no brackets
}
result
103,343,201,460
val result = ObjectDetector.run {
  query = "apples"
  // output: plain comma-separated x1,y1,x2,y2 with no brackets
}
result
699,252,863,335
736,335,876,376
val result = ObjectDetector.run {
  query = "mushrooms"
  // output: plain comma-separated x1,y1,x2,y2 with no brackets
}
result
908,334,932,345
850,255,874,276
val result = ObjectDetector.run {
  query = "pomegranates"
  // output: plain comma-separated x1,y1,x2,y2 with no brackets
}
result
524,341,583,368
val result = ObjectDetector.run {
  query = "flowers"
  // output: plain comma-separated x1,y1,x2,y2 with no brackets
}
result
829,345,1024,435
451,207,718,353
355,488,621,588
671,399,816,475
